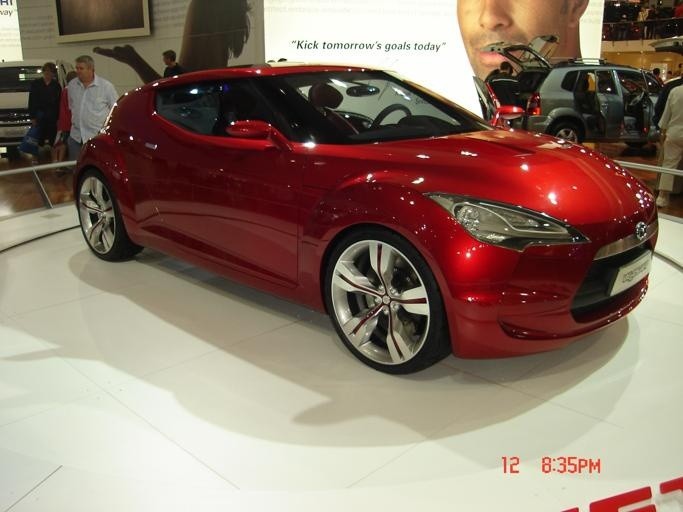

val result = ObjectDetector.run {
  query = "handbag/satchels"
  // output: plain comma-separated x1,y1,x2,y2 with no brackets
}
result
21,127,40,155
50,131,67,162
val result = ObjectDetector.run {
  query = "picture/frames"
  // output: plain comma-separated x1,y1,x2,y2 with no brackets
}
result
51,1,151,44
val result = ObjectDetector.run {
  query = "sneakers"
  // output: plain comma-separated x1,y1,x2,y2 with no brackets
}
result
656,196,669,207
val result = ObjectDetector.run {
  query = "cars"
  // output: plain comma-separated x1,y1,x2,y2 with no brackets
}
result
73,61,661,375
2,59,76,148
471,42,662,145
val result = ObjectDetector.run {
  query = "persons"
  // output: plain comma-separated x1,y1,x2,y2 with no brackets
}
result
67,54,119,171
478,66,514,121
92,0,253,87
456,0,589,81
26,62,61,167
651,63,683,208
604,0,683,40
55,70,77,173
489,62,522,106
162,49,187,77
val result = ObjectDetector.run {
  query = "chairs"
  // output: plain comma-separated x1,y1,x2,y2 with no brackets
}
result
308,82,373,131
575,79,636,130
473,76,526,129
